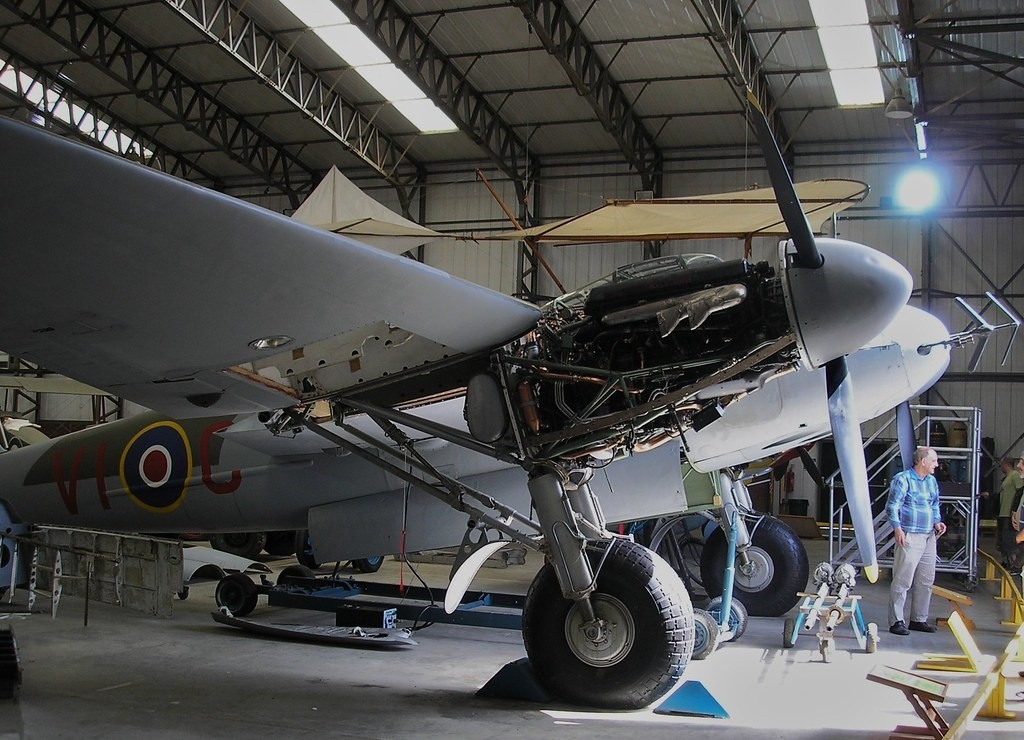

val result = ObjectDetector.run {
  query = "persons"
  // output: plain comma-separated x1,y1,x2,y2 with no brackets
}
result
1010,449,1024,557
885,447,946,635
979,457,1023,575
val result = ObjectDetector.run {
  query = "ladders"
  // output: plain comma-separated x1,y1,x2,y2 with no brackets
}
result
393,548,528,570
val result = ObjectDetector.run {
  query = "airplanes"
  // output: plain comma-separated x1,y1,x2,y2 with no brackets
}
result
207,291,1020,618
1,115,913,709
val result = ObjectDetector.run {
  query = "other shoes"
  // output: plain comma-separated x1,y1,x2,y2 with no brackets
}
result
1011,560,1022,576
908,621,936,632
890,621,909,634
1001,559,1014,569
